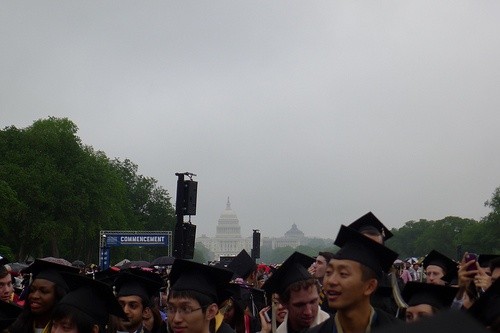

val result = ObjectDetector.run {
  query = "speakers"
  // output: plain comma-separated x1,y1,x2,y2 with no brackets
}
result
181,223,196,260
182,181,197,215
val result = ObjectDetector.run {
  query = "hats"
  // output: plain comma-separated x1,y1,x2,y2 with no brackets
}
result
422,250,460,273
478,254,500,267
228,249,255,280
59,271,130,329
85,267,168,299
347,212,394,241
20,258,81,294
167,258,234,304
229,283,251,310
332,224,399,278
392,281,461,312
260,251,317,299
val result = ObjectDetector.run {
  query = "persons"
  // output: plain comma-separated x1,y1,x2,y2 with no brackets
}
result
0,211,500,332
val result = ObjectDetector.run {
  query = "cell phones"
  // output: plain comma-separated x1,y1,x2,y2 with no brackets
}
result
263,307,273,323
465,254,477,278
